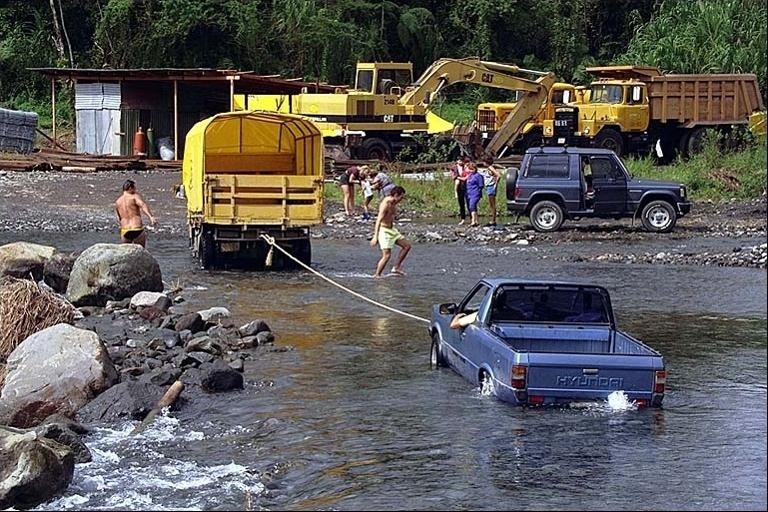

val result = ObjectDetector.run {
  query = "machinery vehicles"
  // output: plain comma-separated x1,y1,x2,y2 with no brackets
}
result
292,51,559,180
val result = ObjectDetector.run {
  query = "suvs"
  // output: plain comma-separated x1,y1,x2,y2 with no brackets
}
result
499,143,692,234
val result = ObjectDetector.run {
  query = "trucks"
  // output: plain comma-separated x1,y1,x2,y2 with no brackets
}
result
470,80,595,139
175,108,325,271
569,64,765,162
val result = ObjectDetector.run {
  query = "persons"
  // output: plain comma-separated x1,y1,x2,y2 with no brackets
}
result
451,156,500,226
339,155,395,216
114,179,160,247
369,186,411,276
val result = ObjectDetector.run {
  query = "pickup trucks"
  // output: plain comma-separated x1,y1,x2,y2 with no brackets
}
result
420,273,671,413
